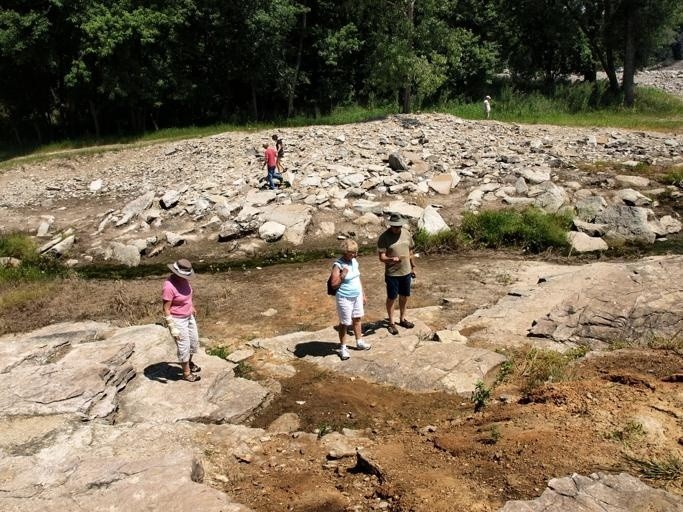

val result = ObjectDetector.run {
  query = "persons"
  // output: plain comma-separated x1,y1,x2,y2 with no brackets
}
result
482,95,491,119
376,211,418,335
261,142,283,188
327,239,370,361
161,258,200,382
271,134,285,175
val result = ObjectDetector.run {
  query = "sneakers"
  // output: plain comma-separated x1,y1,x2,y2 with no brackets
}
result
356,342,371,350
190,362,201,372
388,323,398,335
339,349,351,360
184,373,201,382
399,318,414,329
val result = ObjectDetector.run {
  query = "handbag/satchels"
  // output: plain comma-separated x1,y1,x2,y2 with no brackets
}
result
327,261,344,296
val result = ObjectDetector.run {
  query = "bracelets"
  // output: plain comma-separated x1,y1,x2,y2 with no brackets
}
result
340,272,345,280
167,323,173,327
165,316,172,320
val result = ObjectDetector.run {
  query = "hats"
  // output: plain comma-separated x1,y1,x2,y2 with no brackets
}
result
167,258,196,281
387,212,405,226
